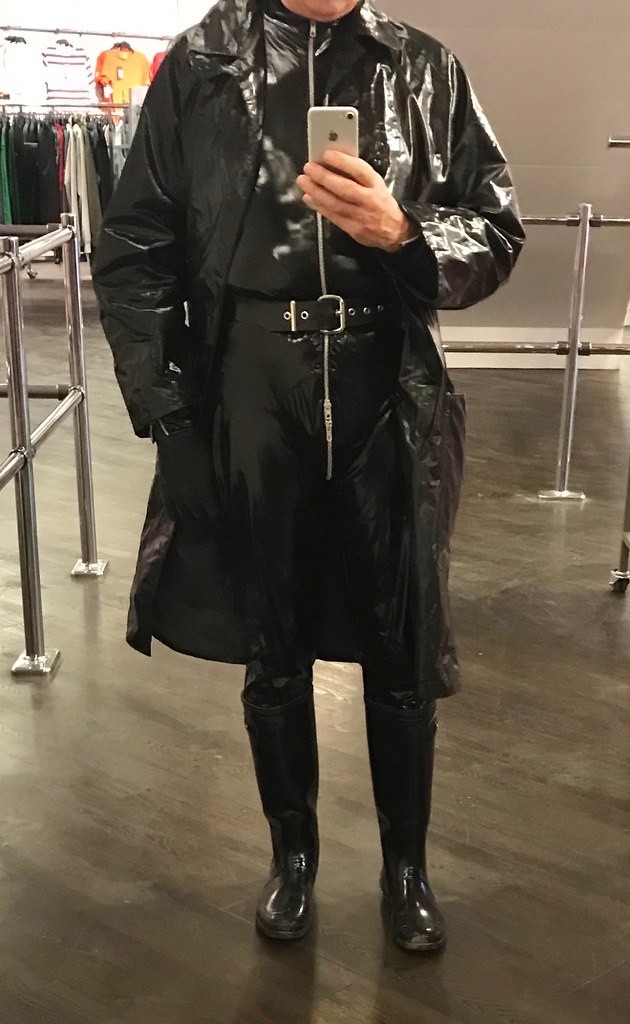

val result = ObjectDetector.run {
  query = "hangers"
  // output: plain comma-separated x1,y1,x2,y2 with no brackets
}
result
0,27,173,54
0,111,115,128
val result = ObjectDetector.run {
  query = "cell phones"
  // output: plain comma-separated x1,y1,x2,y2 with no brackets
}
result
307,106,359,168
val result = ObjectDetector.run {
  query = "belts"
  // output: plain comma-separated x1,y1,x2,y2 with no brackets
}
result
224,291,405,333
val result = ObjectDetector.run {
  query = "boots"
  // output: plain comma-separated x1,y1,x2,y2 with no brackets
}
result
363,694,449,952
240,684,320,941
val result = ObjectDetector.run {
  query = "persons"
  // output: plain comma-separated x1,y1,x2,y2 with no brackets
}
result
89,0,534,952
